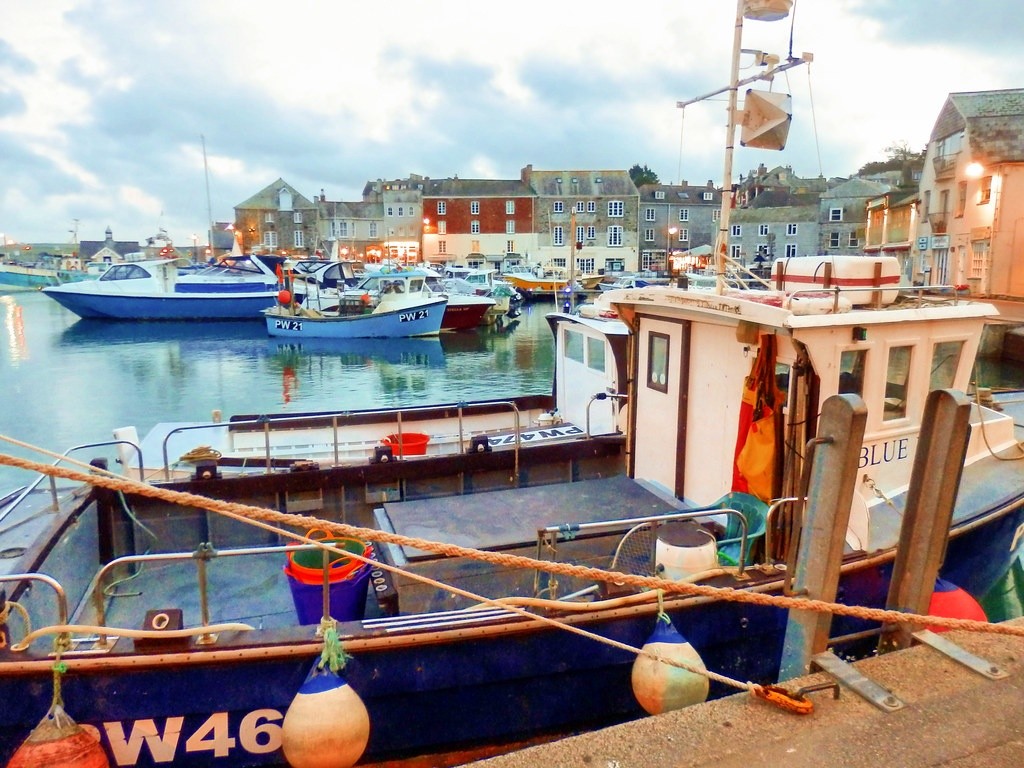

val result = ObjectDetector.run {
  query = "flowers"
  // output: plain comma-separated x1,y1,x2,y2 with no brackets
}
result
954,284,970,290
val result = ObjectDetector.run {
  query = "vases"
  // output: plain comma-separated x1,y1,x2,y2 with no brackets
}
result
957,289,970,295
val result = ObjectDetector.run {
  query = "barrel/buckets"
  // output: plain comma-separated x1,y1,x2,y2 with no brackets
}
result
380,433,430,456
656,523,719,581
283,528,375,626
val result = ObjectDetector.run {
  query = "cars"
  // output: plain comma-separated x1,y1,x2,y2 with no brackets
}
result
2,260,36,268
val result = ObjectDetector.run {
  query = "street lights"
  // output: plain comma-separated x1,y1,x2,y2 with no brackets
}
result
190,235,197,259
668,227,677,256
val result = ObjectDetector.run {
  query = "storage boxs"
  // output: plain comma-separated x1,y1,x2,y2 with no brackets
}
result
770,255,901,304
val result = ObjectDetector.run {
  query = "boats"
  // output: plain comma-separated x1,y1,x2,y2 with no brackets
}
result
259,272,448,337
0,1,1023,768
39,257,746,332
112,306,638,480
